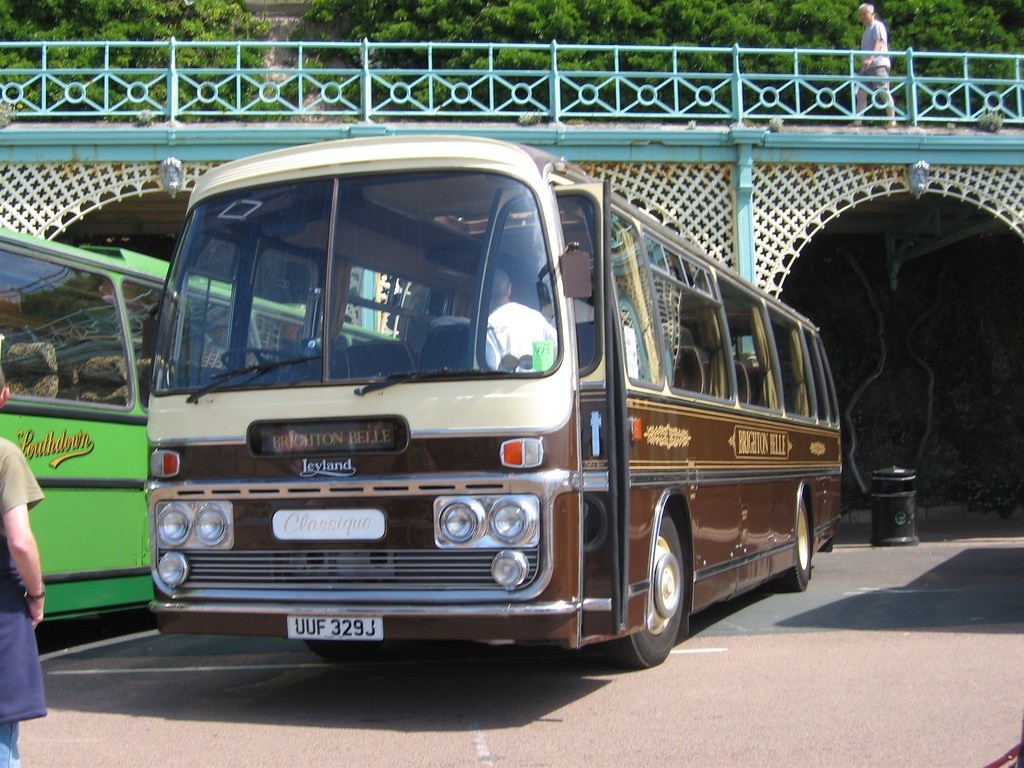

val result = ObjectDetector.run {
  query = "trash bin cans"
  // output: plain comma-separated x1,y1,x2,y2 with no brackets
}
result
870,468,920,546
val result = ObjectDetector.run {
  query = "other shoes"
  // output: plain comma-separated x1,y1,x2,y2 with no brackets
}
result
884,121,899,129
847,122,865,127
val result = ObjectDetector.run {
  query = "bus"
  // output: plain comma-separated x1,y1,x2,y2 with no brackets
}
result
142,134,844,674
0,226,400,620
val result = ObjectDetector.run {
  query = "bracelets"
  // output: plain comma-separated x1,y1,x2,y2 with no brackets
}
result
22,585,46,605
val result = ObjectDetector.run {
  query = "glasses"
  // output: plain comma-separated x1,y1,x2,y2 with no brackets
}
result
497,352,532,374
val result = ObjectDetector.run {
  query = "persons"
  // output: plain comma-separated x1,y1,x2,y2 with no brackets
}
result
0,369,47,768
482,264,557,371
848,4,895,131
